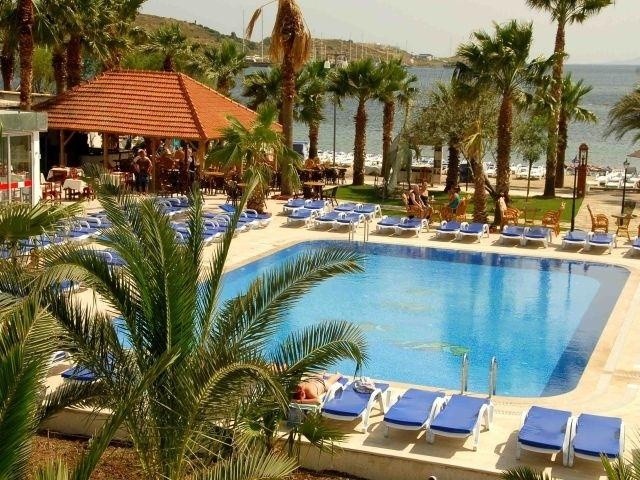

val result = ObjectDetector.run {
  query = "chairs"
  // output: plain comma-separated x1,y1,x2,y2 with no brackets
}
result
2,156,272,296
286,374,350,427
63,353,127,388
319,379,389,435
515,406,573,467
383,387,448,441
569,413,625,467
39,344,73,379
430,395,495,451
272,154,639,257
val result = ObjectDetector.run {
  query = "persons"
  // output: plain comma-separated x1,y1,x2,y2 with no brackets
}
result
445,185,460,215
420,181,433,219
290,367,344,405
105,140,326,196
407,182,428,220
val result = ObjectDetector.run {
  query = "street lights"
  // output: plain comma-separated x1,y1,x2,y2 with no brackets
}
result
620,158,630,225
571,155,580,230
324,53,348,164
